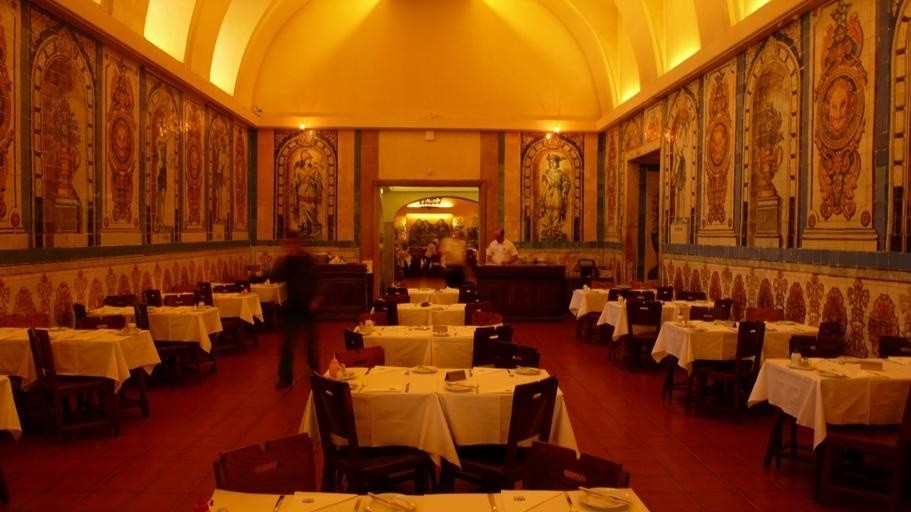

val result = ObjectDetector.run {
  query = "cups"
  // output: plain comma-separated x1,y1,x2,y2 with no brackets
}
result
127,322,136,334
197,301,205,311
617,296,623,305
790,352,802,367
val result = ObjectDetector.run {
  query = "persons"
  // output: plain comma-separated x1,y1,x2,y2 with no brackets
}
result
392,236,440,270
483,227,519,266
261,231,327,389
537,154,574,236
437,225,469,288
290,150,324,236
113,123,129,159
823,77,857,139
672,117,689,224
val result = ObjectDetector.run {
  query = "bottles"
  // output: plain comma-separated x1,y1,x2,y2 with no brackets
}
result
328,354,340,379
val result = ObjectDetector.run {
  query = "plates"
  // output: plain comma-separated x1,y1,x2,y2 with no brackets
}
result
814,368,849,378
786,363,814,370
410,366,438,374
577,487,632,511
513,368,541,375
364,493,415,512
443,382,474,392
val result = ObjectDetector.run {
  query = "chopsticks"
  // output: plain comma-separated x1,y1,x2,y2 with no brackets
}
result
366,492,412,512
578,487,632,505
